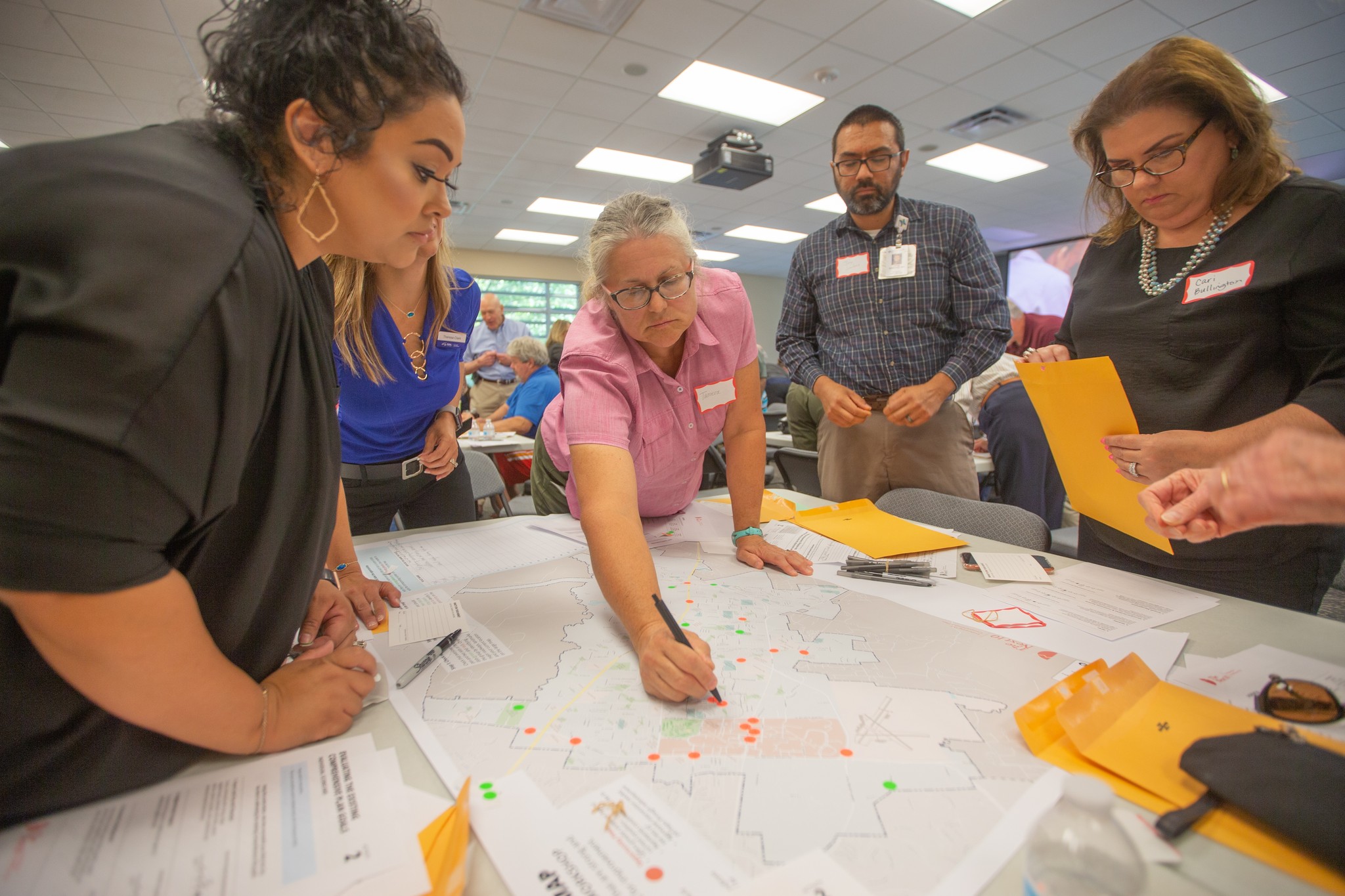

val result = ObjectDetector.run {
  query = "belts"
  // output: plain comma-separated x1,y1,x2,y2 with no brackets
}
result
338,456,425,481
862,394,891,412
980,377,1021,409
479,377,517,384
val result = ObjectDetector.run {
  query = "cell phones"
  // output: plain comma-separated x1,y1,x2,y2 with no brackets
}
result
960,552,1055,574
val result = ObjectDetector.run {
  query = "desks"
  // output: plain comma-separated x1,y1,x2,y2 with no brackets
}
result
458,434,536,517
0,486,1345,896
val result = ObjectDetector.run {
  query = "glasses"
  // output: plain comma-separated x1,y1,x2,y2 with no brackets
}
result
1255,673,1345,724
1095,115,1212,188
598,258,694,312
831,150,903,177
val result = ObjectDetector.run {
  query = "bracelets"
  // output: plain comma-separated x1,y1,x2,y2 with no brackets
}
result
319,567,342,591
330,561,359,572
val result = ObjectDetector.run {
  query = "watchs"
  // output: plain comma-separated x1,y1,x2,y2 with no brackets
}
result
434,404,463,431
731,527,765,547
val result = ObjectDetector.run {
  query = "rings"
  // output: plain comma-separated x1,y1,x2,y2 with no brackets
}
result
449,458,458,468
1220,469,1232,491
906,414,914,423
1127,462,1139,477
353,640,367,649
1022,347,1039,361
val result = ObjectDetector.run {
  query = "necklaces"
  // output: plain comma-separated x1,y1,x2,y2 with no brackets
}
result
255,686,268,754
1138,202,1235,296
369,276,430,319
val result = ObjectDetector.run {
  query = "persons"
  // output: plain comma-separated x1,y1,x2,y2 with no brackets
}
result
699,296,1066,532
329,208,482,538
1137,423,1345,545
772,103,1011,507
0,0,467,834
462,292,574,520
530,191,813,702
1019,33,1345,617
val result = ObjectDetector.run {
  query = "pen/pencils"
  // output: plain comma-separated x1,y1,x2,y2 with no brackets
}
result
841,556,937,586
837,571,933,587
652,594,722,703
396,629,462,689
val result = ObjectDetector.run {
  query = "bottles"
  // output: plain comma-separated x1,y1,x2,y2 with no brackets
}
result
483,419,495,440
468,420,480,441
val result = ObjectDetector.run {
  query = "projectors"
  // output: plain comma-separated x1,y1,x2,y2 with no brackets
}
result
692,148,774,190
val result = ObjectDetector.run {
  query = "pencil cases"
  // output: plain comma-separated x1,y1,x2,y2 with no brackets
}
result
1156,725,1345,874
